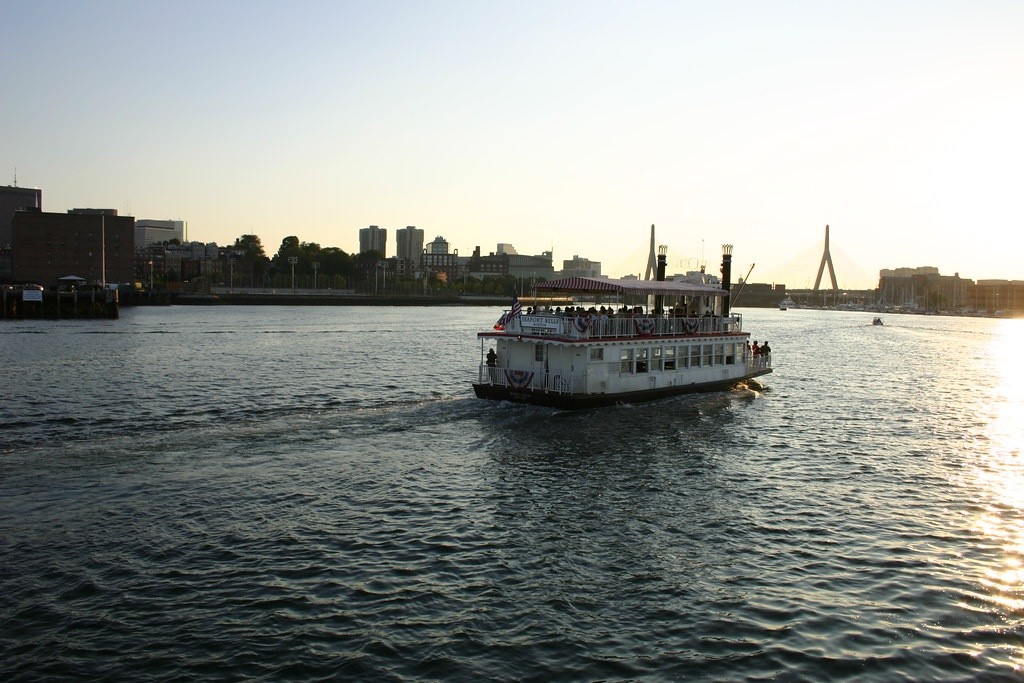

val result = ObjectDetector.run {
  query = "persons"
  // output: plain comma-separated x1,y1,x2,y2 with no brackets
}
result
486,348,497,380
550,306,613,319
752,341,760,359
531,306,538,315
761,341,771,357
668,309,719,324
619,305,643,319
527,307,532,314
743,340,751,362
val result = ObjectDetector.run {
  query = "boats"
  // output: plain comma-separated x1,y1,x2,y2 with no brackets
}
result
472,243,772,409
778,300,787,310
874,317,883,326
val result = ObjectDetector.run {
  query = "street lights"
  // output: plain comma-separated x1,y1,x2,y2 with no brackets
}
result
101,211,105,286
288,256,298,288
312,262,320,288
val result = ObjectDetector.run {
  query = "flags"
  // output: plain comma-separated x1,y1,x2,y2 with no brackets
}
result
497,291,521,325
